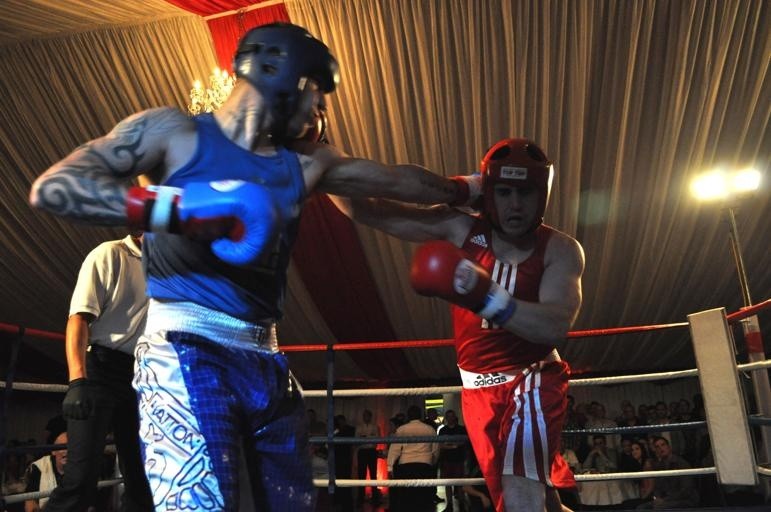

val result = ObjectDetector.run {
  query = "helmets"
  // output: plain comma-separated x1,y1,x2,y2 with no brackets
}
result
233,22,341,122
481,138,556,222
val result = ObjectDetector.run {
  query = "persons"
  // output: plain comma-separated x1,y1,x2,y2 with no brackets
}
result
29,20,483,511
24,400,128,512
65,227,144,505
306,404,496,511
562,394,712,512
324,137,586,512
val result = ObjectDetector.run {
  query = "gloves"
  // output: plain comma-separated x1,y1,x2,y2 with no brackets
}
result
409,238,517,326
280,107,331,154
449,173,485,212
127,178,275,267
62,377,94,419
